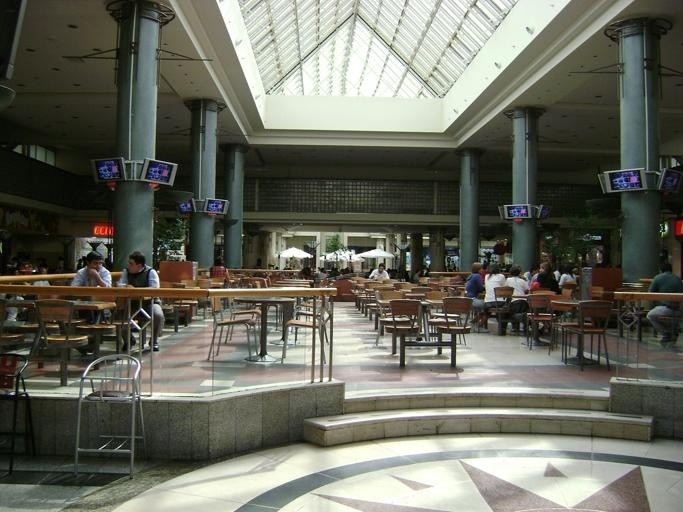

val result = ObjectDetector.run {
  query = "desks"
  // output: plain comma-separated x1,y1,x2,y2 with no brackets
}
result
550,298,598,364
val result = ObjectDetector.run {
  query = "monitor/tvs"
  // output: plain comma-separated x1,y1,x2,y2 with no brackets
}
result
657,168,683,193
503,204,532,219
498,206,504,219
535,205,552,219
91,157,127,183
203,198,228,214
604,168,648,194
139,158,178,186
176,198,196,213
597,174,606,193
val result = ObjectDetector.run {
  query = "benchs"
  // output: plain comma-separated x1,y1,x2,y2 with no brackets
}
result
385,296,475,369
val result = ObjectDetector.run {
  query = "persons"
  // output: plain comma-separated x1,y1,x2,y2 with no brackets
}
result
4,269,32,321
365,264,431,282
445,259,459,271
33,267,51,288
76,258,82,270
58,255,64,270
114,251,165,353
38,263,48,274
646,261,682,344
209,255,229,282
462,259,579,337
274,264,349,279
7,257,19,270
368,262,390,281
81,255,87,268
69,250,112,355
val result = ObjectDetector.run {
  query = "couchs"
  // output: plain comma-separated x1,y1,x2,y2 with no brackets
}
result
328,275,357,302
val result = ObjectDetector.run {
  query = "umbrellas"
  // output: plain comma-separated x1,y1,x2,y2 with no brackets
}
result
318,248,365,271
273,245,314,264
353,248,395,269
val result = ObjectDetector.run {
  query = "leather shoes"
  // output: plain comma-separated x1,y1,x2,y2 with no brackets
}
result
149,343,158,351
123,337,135,350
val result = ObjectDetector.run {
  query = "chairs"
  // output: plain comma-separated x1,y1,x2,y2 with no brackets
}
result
0,355,35,473
564,299,612,373
350,272,487,356
0,270,331,367
74,355,146,480
550,293,595,361
485,270,683,350
